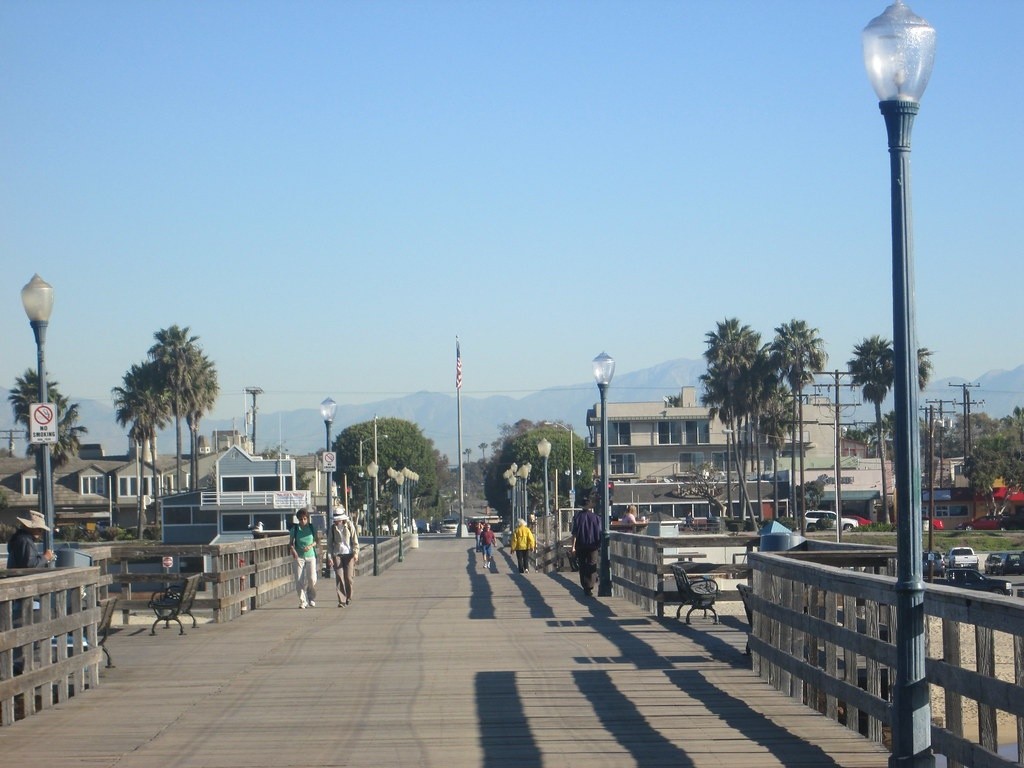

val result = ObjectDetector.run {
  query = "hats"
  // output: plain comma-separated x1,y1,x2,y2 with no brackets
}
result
578,499,595,509
330,508,349,522
17,509,51,532
516,519,527,527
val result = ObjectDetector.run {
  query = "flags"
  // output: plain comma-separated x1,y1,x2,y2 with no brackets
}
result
454,344,463,390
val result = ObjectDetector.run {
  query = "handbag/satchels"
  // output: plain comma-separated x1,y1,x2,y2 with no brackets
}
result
570,552,581,571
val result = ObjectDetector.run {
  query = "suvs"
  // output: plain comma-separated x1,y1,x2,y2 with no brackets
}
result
805,509,858,532
922,551,945,579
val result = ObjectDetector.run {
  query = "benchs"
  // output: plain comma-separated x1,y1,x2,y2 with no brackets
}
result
12,596,117,695
148,574,200,636
736,582,895,730
669,563,722,625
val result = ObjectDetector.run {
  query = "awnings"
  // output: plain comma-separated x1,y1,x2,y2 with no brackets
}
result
921,490,952,501
992,487,1024,501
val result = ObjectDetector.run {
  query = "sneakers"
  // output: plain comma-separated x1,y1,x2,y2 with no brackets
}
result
309,600,315,606
299,600,308,609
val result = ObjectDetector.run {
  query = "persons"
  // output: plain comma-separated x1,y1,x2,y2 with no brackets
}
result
475,523,485,552
570,498,602,597
685,512,694,531
327,506,359,607
95,521,100,529
509,518,535,573
479,524,496,568
623,504,636,534
4,508,54,610
288,507,320,609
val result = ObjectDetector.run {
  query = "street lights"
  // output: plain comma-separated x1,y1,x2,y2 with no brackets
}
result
319,396,338,578
386,465,419,562
367,460,379,576
537,437,552,573
592,350,616,597
358,434,388,468
858,0,949,768
545,421,574,532
722,429,805,536
502,462,533,537
20,273,56,570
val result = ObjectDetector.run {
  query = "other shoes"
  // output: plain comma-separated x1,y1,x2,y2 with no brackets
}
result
520,569,530,575
483,564,490,568
583,587,593,595
346,597,351,605
338,603,346,608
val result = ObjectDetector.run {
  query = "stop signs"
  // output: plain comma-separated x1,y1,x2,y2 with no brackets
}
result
164,558,173,567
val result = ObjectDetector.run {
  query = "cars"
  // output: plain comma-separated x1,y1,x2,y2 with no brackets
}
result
956,509,1024,531
440,519,458,534
843,515,871,527
922,516,944,530
984,550,1024,576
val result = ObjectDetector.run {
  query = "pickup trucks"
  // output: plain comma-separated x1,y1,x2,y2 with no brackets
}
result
922,568,1013,597
948,547,979,572
